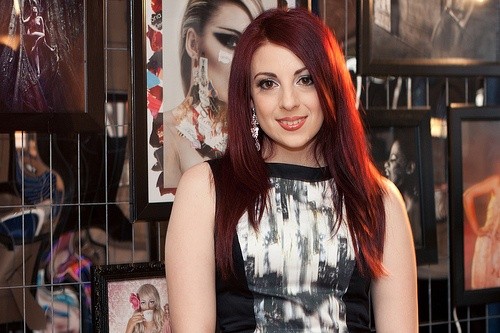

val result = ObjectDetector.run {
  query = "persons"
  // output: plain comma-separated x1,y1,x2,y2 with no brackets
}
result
463,172,500,288
20,6,48,79
385,135,426,250
164,0,265,189
165,6,419,333
126,284,172,333
430,0,476,57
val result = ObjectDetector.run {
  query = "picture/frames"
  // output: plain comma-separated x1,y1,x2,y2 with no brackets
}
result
359,106,439,266
126,0,308,222
356,0,500,78
90,260,171,333
0,0,105,136
447,103,500,307
